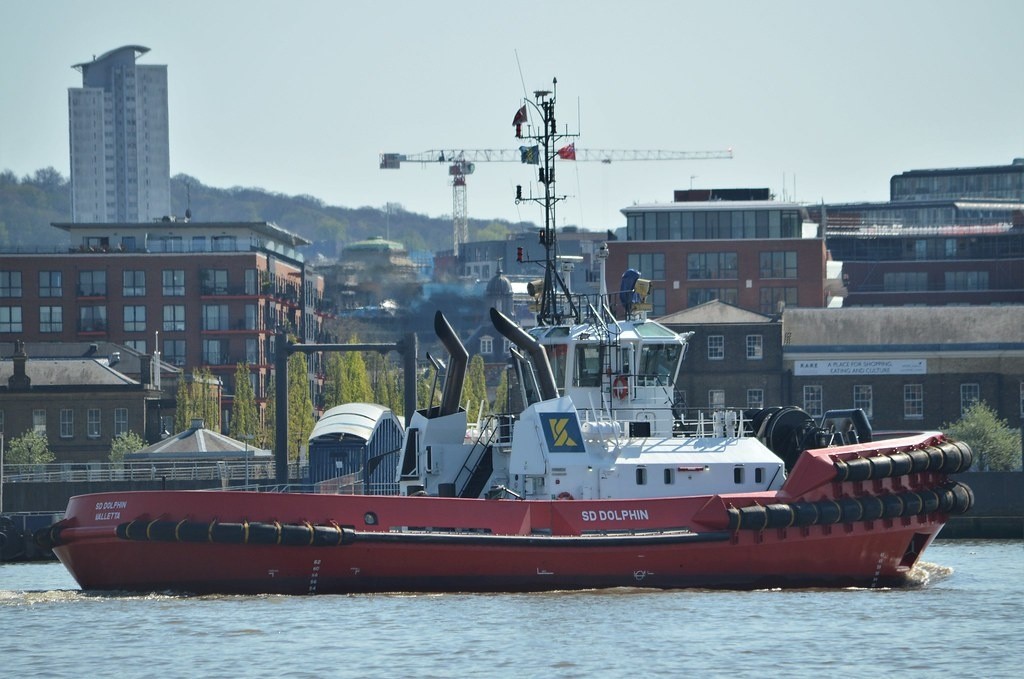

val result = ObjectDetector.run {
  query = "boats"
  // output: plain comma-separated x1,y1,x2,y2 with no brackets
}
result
35,50,973,592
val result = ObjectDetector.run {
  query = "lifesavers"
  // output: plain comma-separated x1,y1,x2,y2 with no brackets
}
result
613,377,626,399
556,492,574,500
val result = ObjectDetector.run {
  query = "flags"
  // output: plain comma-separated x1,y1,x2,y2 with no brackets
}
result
519,145,540,164
558,143,576,160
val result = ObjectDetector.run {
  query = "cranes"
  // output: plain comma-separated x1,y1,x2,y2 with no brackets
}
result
377,147,734,260
